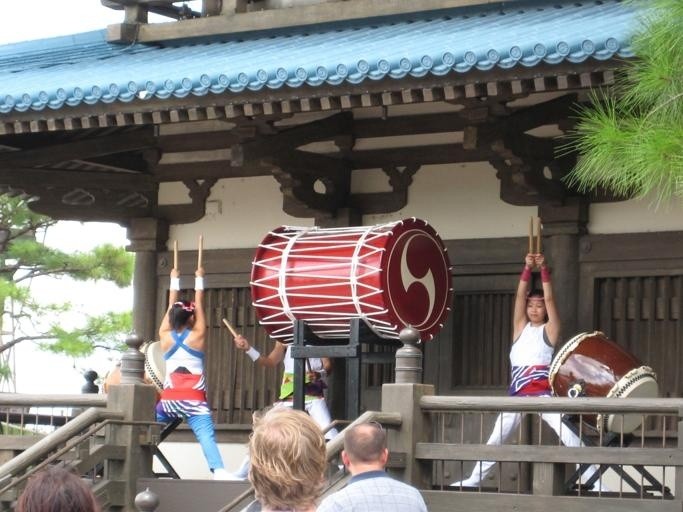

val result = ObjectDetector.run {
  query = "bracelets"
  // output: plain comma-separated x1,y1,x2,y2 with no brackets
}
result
167,276,181,292
192,275,205,292
243,345,261,364
538,265,553,283
518,266,532,283
317,367,329,380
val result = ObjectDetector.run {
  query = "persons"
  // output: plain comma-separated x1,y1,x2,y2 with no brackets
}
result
232,330,342,481
449,251,613,495
314,418,430,512
244,400,330,512
152,265,240,481
12,463,103,512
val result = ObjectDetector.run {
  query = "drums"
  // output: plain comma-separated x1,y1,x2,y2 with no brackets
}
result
549,330,660,433
101,340,166,400
250,217,453,345
